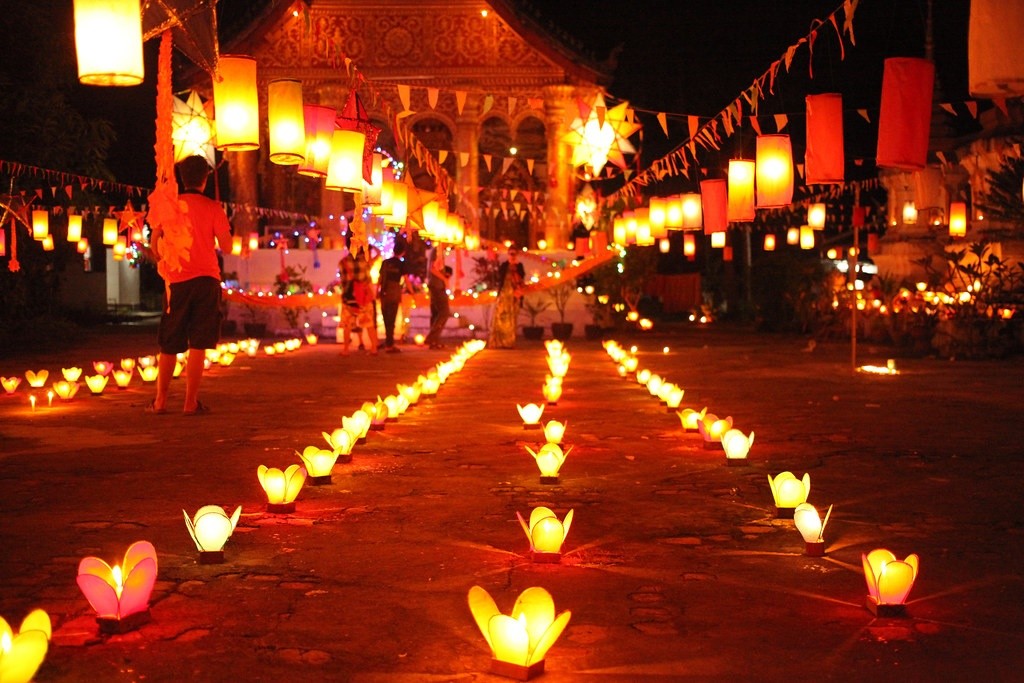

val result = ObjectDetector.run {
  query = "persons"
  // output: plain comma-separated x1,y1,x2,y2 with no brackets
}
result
146,154,233,415
337,233,525,352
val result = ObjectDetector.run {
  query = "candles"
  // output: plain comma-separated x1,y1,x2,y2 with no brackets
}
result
0,331,922,683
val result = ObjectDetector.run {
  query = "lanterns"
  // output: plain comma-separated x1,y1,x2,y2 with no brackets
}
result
0,0,1024,268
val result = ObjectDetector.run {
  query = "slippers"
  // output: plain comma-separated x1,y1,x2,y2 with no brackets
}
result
188,403,209,415
146,400,164,413
385,346,401,353
